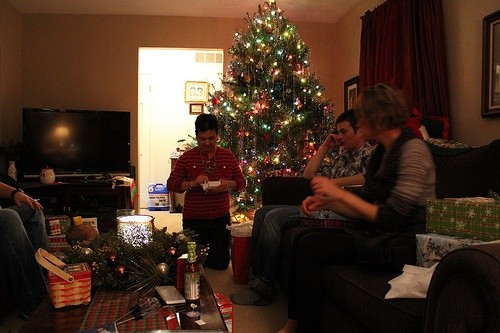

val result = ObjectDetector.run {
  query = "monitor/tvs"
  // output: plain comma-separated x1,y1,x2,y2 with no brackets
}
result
22,106,131,178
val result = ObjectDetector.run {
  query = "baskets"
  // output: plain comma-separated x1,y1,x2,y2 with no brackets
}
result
34,248,92,309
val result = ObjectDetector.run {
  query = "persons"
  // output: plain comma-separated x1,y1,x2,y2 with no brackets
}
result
0,179,49,318
167,113,246,271
227,106,378,305
277,82,437,333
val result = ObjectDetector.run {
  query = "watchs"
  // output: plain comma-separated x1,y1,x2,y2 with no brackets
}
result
10,188,24,203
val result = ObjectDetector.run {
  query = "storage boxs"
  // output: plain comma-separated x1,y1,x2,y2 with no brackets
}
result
425,197,500,241
215,290,235,333
416,232,500,268
297,202,346,230
45,214,71,257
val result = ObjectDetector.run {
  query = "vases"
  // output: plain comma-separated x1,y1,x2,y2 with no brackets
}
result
8,161,18,181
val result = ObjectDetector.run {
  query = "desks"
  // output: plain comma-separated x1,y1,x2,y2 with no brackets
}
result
170,156,177,212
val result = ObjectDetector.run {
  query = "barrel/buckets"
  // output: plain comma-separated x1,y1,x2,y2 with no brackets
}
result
148,183,169,211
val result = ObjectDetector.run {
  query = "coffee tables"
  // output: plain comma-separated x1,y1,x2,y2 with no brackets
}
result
14,265,229,333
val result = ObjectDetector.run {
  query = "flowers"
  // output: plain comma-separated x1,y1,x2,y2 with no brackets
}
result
0,139,23,160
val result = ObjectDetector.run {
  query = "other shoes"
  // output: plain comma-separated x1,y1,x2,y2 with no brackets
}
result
230,286,274,306
18,302,38,320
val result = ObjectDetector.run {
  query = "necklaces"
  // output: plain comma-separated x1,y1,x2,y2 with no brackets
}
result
199,150,216,174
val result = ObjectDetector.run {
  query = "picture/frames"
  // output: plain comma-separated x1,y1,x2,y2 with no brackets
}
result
481,9,500,117
343,76,360,111
190,103,204,115
184,81,210,103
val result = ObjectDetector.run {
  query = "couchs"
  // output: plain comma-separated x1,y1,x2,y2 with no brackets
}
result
262,138,500,333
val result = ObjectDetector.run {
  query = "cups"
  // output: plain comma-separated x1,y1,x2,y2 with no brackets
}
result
116,209,136,216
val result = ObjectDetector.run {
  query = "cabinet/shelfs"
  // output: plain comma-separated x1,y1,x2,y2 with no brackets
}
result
15,181,129,232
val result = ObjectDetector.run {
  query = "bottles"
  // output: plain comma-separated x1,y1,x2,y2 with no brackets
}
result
8,161,17,182
184,242,201,306
49,219,61,235
40,166,55,184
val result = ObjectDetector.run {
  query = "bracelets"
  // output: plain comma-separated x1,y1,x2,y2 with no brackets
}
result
186,181,192,190
